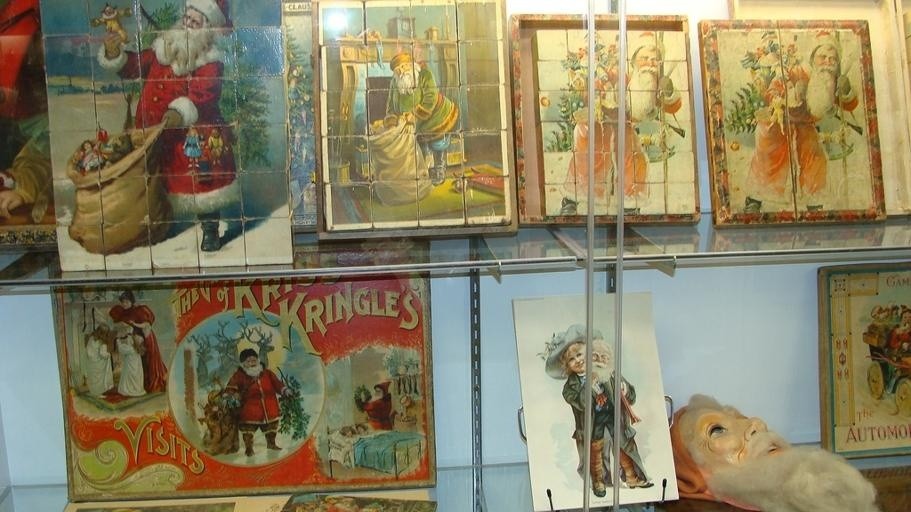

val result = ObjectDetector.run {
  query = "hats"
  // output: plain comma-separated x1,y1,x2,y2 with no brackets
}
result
808,31,842,65
390,53,413,71
119,290,136,304
374,381,392,394
239,348,258,363
544,323,603,379
630,32,666,67
183,0,235,36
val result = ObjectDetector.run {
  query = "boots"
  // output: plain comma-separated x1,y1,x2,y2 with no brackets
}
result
431,151,450,184
198,212,221,251
243,433,254,457
587,440,654,497
265,432,283,451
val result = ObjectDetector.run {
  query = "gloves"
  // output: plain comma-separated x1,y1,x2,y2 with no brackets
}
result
838,75,851,96
161,108,182,132
105,33,120,59
659,76,673,98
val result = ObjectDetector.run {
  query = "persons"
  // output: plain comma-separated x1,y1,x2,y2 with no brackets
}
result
342,426,375,458
389,50,460,186
84,324,117,402
224,347,295,455
667,390,884,512
536,322,656,497
0,130,51,219
352,422,372,438
110,320,148,397
180,127,206,170
555,43,684,215
92,4,135,45
575,337,658,482
208,126,225,166
361,381,396,431
868,300,911,372
108,289,167,393
82,140,103,173
89,1,248,256
740,28,860,211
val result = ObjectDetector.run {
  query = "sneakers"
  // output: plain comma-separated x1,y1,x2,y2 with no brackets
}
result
557,202,578,216
742,199,760,214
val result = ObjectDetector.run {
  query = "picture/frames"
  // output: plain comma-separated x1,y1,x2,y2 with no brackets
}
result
43,241,439,501
816,260,911,460
314,1,520,243
694,16,891,229
505,12,704,229
